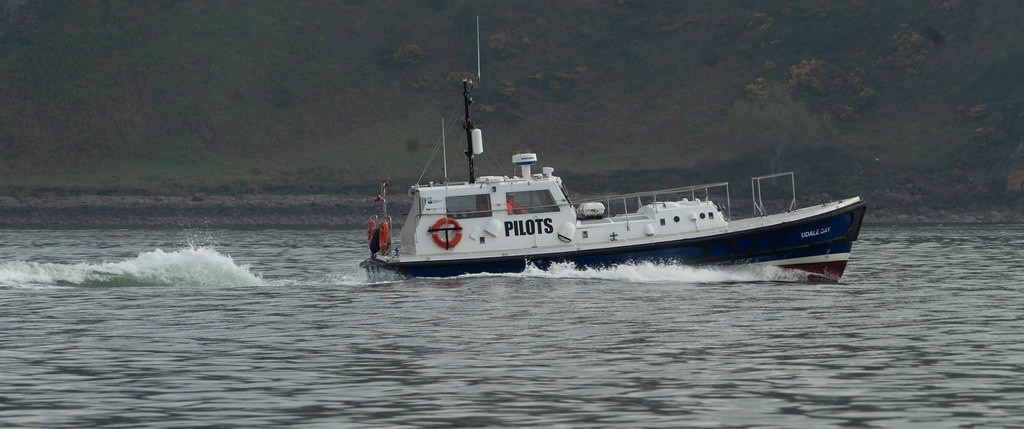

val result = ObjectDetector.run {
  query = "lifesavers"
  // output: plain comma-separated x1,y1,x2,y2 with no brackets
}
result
365,220,390,253
431,218,462,248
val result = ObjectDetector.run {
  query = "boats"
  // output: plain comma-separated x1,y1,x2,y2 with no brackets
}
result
358,13,867,288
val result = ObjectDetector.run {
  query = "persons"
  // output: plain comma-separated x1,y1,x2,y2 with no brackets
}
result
506,193,532,214
